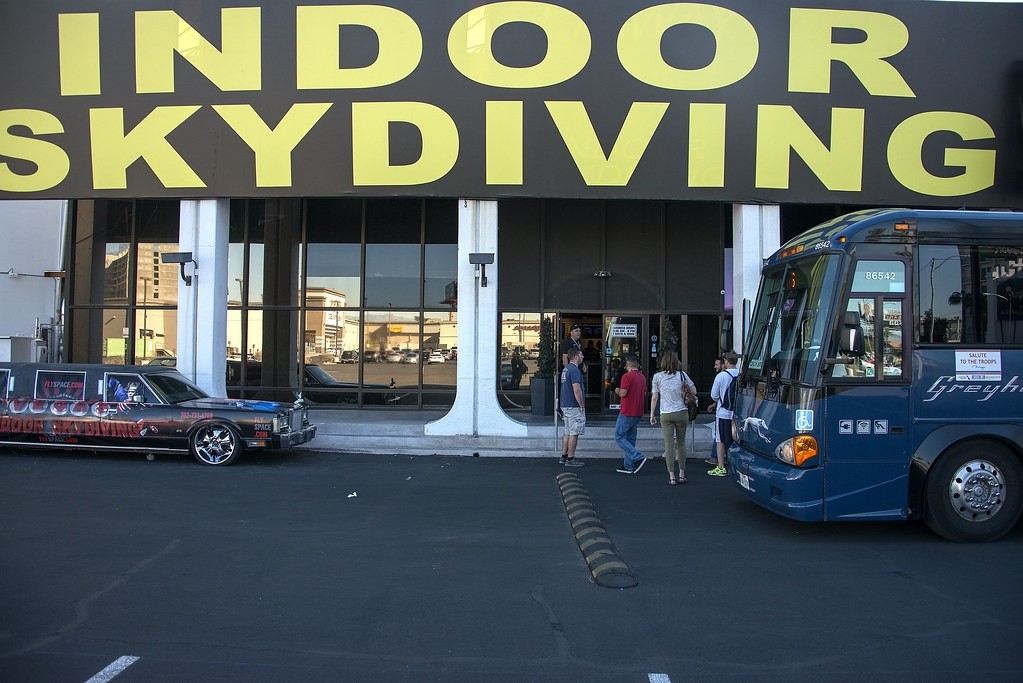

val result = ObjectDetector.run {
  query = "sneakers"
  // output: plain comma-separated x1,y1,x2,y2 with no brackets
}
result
558,457,585,467
707,466,728,476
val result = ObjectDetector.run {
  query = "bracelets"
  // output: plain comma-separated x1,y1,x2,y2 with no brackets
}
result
569,361,578,367
650,415,654,418
617,390,620,394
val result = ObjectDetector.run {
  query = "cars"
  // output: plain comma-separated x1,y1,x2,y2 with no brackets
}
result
433,349,452,360
527,348,539,360
0,362,317,466
502,350,509,360
428,354,445,364
512,349,528,358
423,351,430,360
148,357,391,406
502,363,520,390
340,350,359,364
386,351,419,364
365,350,383,363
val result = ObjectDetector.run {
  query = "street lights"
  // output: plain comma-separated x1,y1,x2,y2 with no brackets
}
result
140,276,151,360
983,292,1011,345
388,302,392,348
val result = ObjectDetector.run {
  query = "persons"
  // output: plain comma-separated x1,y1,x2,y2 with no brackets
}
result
653,424,678,462
559,348,585,466
613,356,647,473
556,325,586,418
586,339,599,363
510,351,523,389
707,351,739,476
704,357,728,464
649,352,696,486
609,358,622,404
861,304,873,321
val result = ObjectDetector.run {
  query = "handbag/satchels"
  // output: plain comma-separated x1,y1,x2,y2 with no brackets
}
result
680,370,698,409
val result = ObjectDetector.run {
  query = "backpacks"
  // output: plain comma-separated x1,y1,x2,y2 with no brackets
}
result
719,370,736,411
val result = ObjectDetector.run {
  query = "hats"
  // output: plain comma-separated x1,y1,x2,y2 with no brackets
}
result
570,324,581,332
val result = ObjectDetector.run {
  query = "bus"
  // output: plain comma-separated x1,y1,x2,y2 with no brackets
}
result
719,208,1023,542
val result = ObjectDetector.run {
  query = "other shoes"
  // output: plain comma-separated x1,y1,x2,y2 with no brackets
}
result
678,477,688,483
633,458,648,474
616,466,633,473
705,459,717,465
669,479,678,485
